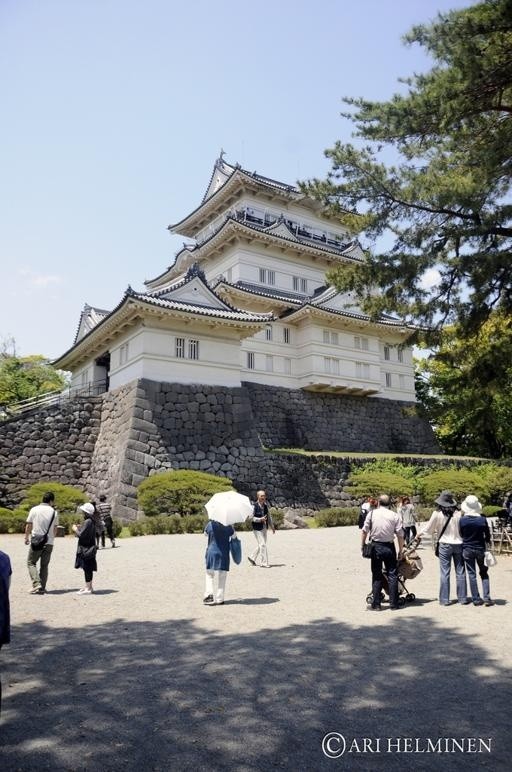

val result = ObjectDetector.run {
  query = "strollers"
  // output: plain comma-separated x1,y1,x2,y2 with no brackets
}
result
365,538,423,607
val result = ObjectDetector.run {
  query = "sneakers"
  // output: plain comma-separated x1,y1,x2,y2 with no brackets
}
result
29,585,46,595
202,595,215,605
76,588,94,595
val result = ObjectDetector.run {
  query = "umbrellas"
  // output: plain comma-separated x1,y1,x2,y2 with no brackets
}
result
203,490,254,528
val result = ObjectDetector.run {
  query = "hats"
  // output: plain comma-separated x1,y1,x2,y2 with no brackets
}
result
435,491,482,513
78,503,95,516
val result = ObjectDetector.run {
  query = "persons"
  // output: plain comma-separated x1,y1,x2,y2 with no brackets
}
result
357,490,497,611
89,500,106,550
247,491,276,568
71,502,98,594
97,494,116,549
203,520,236,606
1,551,14,711
24,491,58,595
506,494,512,525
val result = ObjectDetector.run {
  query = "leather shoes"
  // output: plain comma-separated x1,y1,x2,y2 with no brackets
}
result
485,601,495,606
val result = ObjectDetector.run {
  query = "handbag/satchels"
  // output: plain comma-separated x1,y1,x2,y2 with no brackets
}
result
435,542,439,557
229,539,241,564
483,550,498,567
105,516,113,527
31,535,48,551
363,544,374,558
